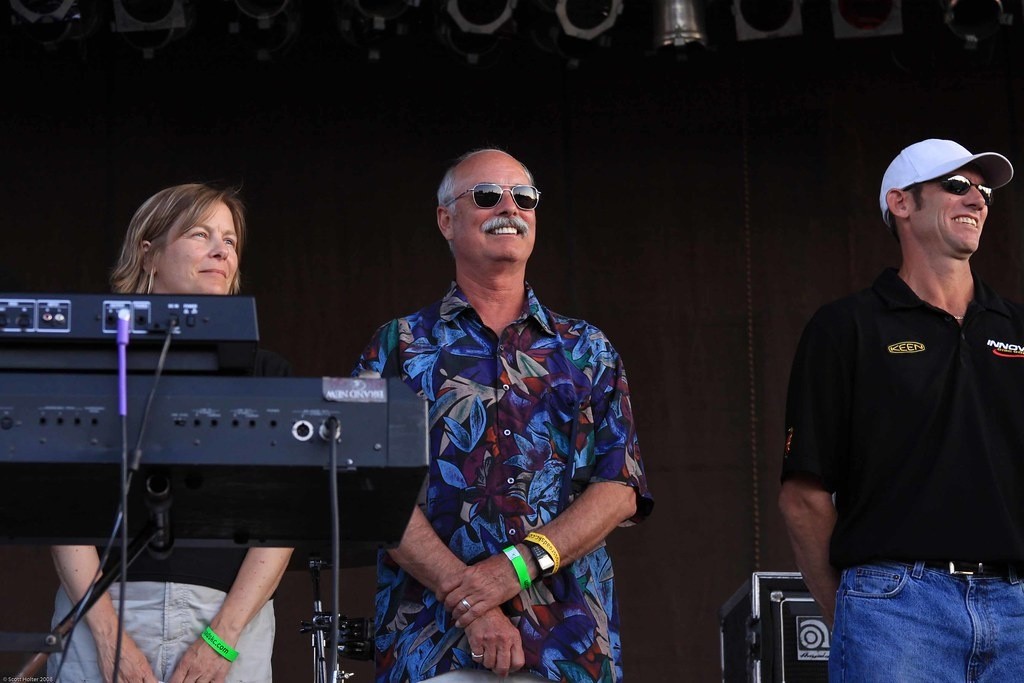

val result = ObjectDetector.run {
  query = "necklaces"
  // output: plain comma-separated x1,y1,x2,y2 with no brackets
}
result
954,316,964,320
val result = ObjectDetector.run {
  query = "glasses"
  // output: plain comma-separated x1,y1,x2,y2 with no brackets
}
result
936,173,995,207
880,138,1014,228
443,182,542,211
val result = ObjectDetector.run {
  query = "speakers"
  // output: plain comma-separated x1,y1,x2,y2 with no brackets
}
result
769,590,831,683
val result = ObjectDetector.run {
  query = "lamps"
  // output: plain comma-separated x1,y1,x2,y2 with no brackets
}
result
0,0,1024,55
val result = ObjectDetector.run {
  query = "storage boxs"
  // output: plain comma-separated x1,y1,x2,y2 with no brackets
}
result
718,572,838,683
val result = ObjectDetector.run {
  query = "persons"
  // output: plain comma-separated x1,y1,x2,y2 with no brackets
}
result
49,185,294,683
354,149,654,683
778,139,1024,683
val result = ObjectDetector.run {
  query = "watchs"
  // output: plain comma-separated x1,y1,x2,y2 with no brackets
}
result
522,541,554,583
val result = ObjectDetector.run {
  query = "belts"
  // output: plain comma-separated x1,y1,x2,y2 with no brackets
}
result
890,557,1005,576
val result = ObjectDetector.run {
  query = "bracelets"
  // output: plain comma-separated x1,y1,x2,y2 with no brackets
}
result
524,532,560,573
503,545,532,590
201,626,239,661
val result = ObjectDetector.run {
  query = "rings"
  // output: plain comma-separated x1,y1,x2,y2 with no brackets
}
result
461,599,471,609
472,652,483,658
